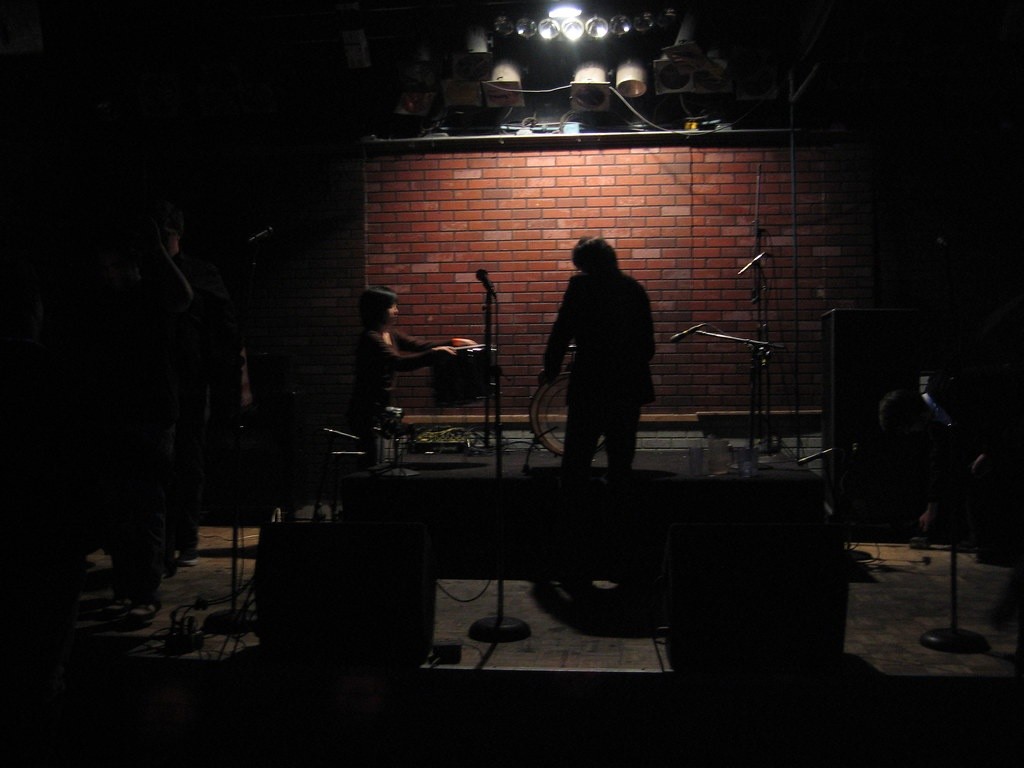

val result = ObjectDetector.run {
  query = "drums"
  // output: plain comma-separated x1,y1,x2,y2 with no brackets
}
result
432,342,496,405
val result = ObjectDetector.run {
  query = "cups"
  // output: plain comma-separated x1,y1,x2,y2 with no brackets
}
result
689,446,703,476
709,439,734,474
738,446,758,477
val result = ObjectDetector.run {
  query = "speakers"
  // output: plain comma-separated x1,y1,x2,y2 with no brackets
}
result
820,306,928,524
202,351,299,526
247,519,441,670
657,518,853,674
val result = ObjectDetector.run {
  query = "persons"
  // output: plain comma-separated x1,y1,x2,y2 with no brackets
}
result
0,193,254,627
346,286,460,436
538,241,656,486
876,364,1024,565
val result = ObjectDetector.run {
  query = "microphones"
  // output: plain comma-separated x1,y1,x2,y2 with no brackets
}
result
669,322,707,345
476,268,495,296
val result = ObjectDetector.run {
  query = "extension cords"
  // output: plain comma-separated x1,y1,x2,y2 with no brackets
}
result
164,628,204,655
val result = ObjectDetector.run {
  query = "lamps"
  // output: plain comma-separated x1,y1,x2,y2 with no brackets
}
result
465,8,677,113
